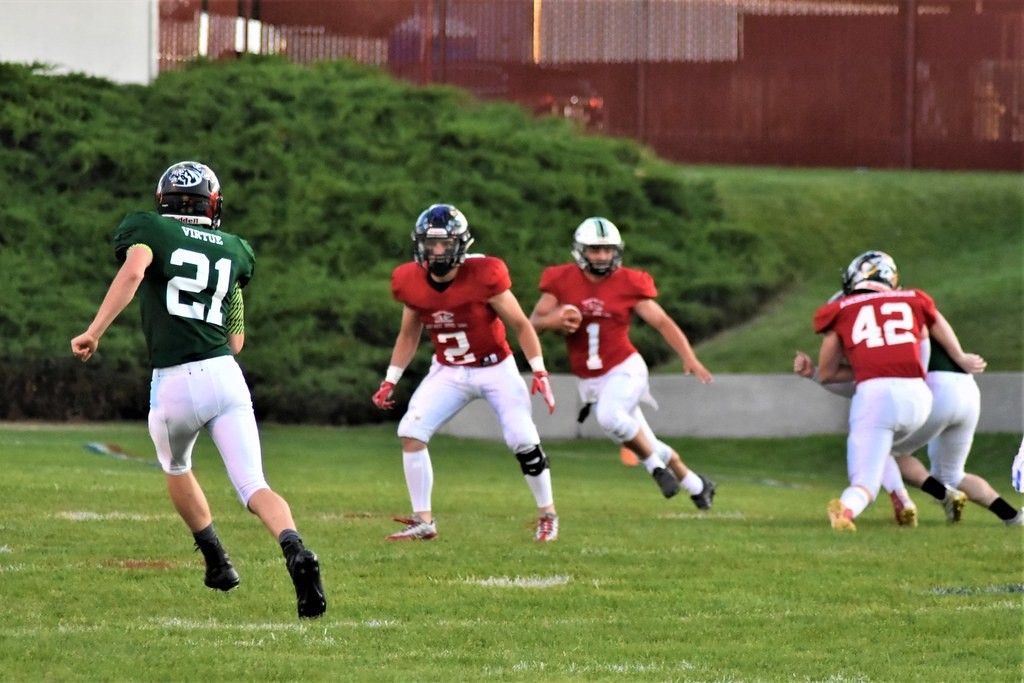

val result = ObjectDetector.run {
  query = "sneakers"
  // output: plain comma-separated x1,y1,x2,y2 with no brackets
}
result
286,550,327,619
385,515,437,543
534,511,559,543
204,553,240,592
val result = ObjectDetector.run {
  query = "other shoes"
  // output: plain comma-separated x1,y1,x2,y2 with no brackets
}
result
826,498,857,534
690,475,717,511
653,465,680,499
1006,510,1024,528
890,489,918,529
936,484,967,523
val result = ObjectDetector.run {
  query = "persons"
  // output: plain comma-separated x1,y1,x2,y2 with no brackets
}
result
817,250,987,533
794,291,1023,525
70,162,329,620
372,205,558,542
531,216,717,512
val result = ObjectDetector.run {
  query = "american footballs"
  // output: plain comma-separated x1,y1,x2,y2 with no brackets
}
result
552,304,582,336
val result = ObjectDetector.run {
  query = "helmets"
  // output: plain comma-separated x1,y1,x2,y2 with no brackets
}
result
411,204,475,277
571,216,626,278
839,250,900,294
155,161,223,229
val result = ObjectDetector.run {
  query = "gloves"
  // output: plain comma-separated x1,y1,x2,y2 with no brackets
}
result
372,380,395,410
531,371,554,414
